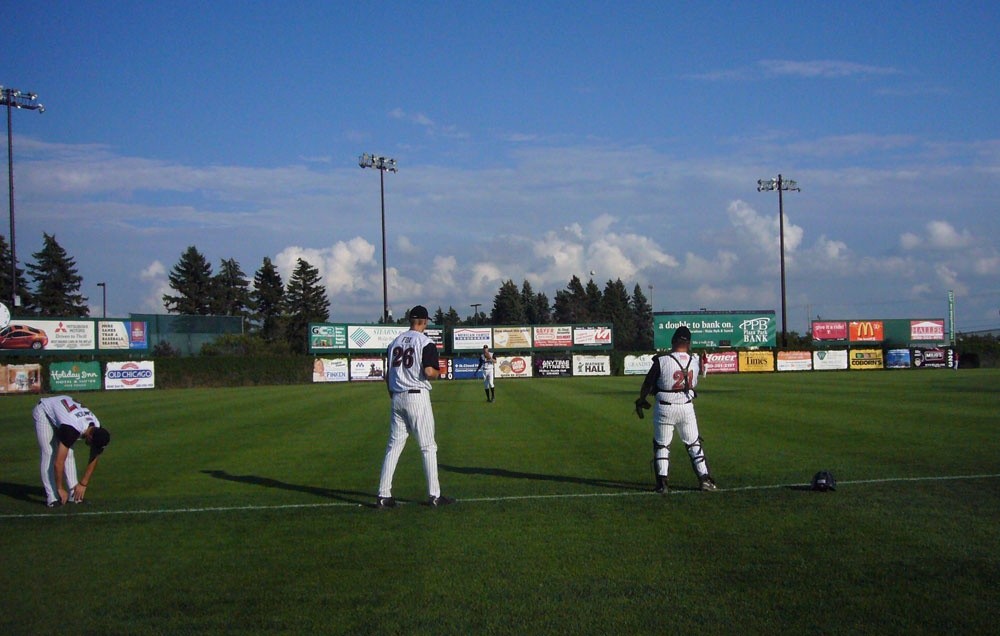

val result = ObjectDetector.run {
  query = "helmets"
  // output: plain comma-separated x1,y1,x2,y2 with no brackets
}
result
809,471,837,492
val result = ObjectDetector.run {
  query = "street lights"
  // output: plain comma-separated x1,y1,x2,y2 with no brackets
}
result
96,282,106,321
357,154,401,327
756,173,802,348
470,303,482,326
647,284,654,313
0,85,49,314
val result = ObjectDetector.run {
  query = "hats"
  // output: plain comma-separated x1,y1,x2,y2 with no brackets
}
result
92,427,110,455
409,305,432,320
484,345,488,348
674,326,691,341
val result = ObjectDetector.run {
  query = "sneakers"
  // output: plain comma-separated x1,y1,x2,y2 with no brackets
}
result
376,494,401,509
654,479,669,494
48,500,61,508
67,496,85,504
427,496,456,507
699,480,719,491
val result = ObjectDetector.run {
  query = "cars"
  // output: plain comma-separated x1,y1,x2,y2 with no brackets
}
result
0,323,50,349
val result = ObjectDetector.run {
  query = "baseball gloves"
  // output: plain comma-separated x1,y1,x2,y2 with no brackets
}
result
484,352,491,360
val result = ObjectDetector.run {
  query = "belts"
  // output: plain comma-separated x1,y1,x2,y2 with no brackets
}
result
660,401,691,405
396,390,420,393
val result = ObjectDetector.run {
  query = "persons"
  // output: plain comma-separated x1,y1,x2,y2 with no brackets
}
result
376,305,457,506
474,345,497,402
635,326,717,492
31,395,110,508
314,360,326,381
702,350,709,378
